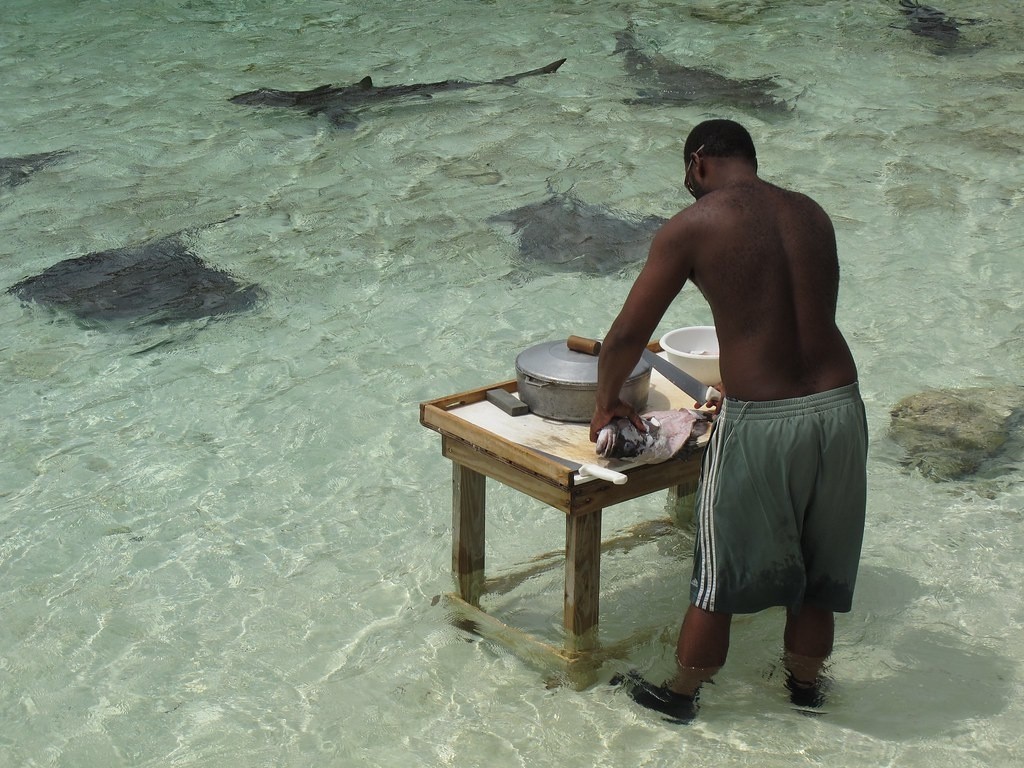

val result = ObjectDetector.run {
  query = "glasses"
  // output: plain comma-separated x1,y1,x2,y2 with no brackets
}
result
684,144,705,197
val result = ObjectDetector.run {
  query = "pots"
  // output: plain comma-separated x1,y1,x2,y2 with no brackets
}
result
515,336,655,423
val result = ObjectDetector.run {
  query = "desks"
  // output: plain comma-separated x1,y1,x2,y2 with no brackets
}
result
438,434,708,693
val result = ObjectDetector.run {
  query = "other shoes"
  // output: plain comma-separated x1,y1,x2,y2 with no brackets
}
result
623,669,699,725
784,668,832,718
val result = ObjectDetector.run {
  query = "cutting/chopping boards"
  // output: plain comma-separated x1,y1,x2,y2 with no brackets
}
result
444,349,717,485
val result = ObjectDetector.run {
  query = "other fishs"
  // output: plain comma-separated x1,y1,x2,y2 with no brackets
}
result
1,1,997,464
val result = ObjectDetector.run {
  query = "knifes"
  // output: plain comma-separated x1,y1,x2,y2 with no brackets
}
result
640,346,724,406
515,443,628,485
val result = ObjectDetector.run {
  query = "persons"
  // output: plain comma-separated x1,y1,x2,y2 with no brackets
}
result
590,119,868,726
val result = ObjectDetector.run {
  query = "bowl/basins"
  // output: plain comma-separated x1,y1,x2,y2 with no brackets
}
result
658,326,721,387
